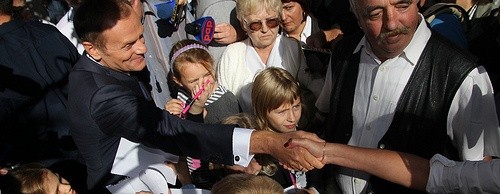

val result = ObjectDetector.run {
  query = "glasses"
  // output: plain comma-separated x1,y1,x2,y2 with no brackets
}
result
244,16,280,32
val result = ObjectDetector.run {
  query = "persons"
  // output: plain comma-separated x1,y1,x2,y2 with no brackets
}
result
0,0,500,194
